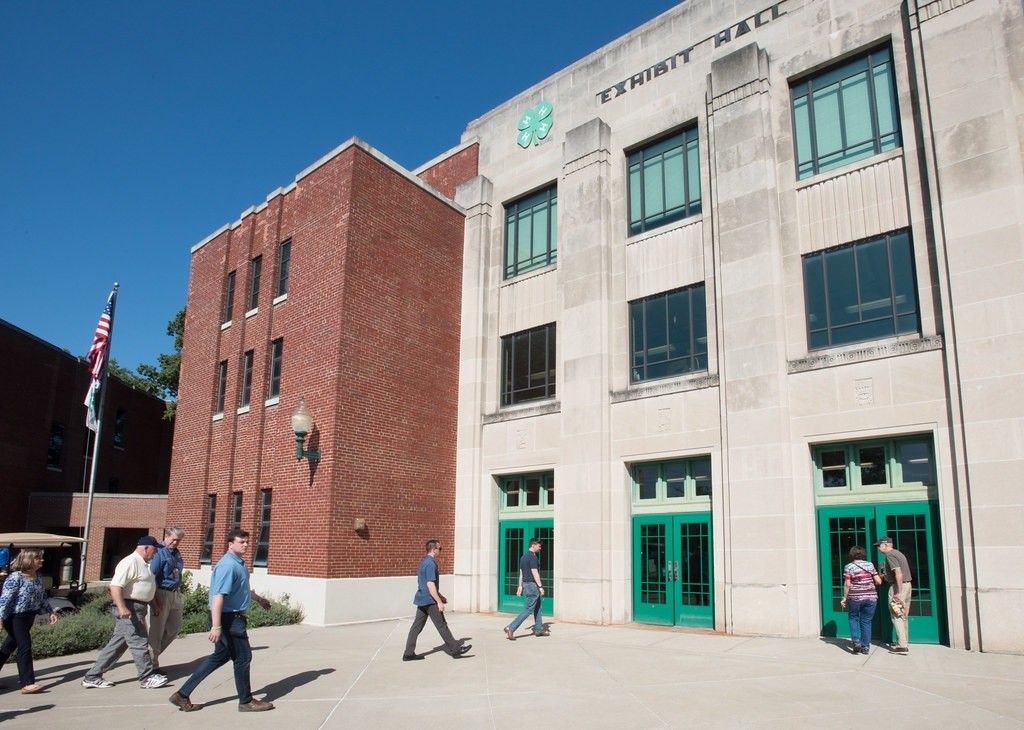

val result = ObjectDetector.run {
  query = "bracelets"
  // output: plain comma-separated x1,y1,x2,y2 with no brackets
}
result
538,586,542,588
211,626,222,630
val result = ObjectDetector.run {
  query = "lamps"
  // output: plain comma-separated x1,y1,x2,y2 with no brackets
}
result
290,396,322,462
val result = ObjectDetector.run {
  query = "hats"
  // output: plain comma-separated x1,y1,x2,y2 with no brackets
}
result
872,536,893,545
138,536,165,548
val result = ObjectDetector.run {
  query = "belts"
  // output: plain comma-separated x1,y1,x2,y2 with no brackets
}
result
890,581,910,585
159,585,177,592
522,582,536,584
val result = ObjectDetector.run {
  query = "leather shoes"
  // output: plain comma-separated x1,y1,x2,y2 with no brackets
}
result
169,692,203,711
535,631,550,636
452,645,472,659
239,698,274,711
403,654,424,660
504,626,516,640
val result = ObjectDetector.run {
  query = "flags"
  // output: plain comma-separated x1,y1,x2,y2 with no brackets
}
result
84,290,114,433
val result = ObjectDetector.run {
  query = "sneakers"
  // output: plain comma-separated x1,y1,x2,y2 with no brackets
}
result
82,677,114,689
141,674,168,688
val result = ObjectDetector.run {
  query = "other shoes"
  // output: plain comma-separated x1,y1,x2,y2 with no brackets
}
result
886,643,897,648
852,642,861,654
23,682,43,693
152,668,166,677
862,645,869,654
888,648,909,656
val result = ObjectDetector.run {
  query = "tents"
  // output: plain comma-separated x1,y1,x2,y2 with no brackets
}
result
0,533,91,589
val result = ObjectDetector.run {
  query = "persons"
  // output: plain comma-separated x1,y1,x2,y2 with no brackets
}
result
403,540,472,661
504,538,550,640
169,530,273,712
873,537,912,655
840,546,884,655
0,548,58,694
81,536,168,689
149,528,184,675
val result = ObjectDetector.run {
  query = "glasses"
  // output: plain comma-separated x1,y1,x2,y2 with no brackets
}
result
436,548,441,551
